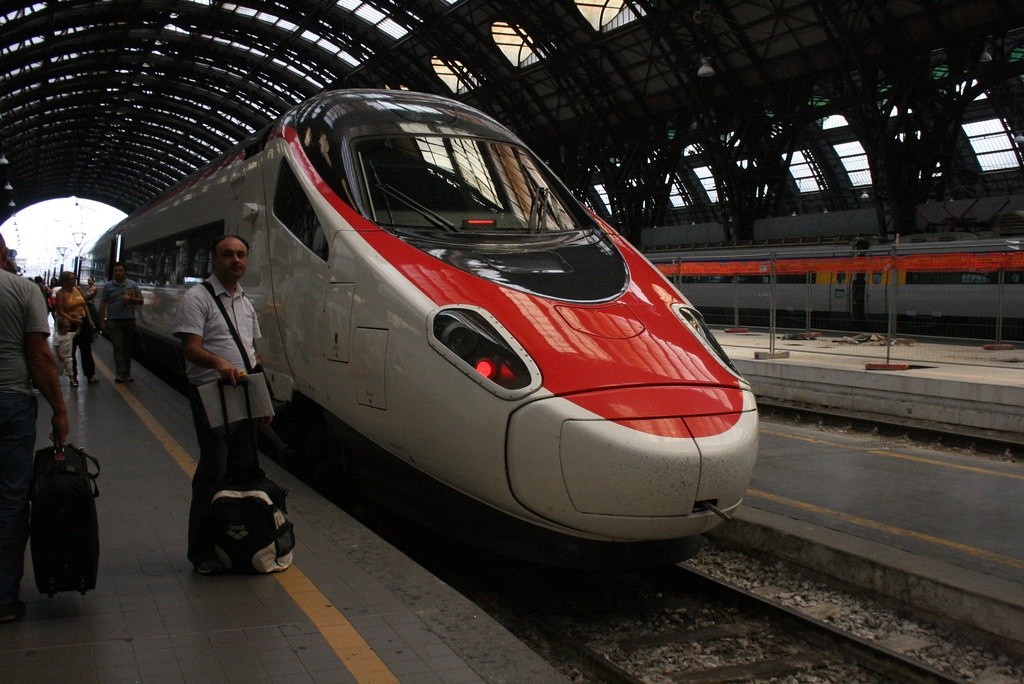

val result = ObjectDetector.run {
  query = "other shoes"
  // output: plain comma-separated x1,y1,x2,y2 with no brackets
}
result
89,379,100,384
126,375,135,382
71,377,80,387
114,375,124,383
189,558,229,578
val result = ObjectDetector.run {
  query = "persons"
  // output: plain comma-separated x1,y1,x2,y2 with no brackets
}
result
99,262,143,383
26,271,100,387
171,235,273,574
0,234,69,623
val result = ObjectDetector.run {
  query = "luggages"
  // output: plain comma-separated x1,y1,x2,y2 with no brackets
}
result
32,430,102,599
198,370,294,572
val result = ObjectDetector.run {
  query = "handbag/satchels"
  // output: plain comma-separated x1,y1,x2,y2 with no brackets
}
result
251,364,274,400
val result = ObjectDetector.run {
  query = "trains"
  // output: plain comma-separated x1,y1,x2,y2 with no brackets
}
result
641,243,1023,335
41,87,760,572
